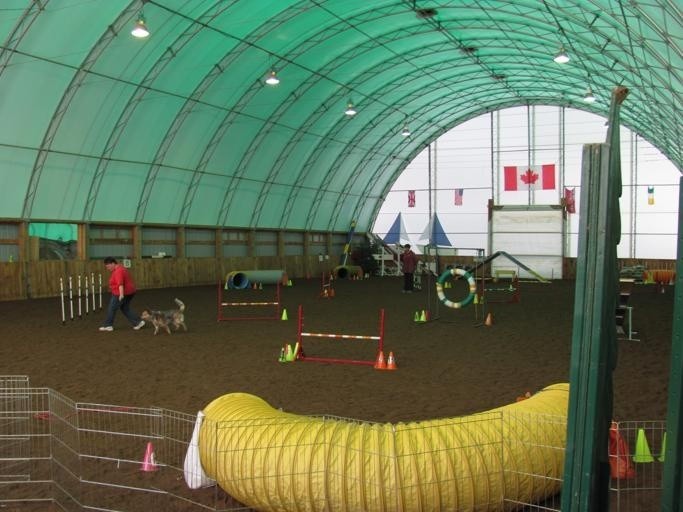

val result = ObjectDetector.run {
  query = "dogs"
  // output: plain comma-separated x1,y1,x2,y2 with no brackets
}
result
139,298,188,336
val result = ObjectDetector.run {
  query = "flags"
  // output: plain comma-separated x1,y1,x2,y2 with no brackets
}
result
564,186,576,214
646,185,654,205
407,189,415,207
454,188,463,206
504,164,556,192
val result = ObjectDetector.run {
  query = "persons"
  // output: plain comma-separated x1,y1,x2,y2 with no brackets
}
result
97,255,146,332
400,243,417,294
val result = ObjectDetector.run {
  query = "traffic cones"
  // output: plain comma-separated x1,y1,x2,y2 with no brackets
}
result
258,281,263,291
658,432,669,463
485,313,493,327
443,281,451,289
276,343,305,364
142,442,161,474
223,282,230,291
353,272,369,281
388,350,399,371
413,304,431,321
321,286,335,298
374,348,387,370
281,308,288,322
251,282,258,291
633,429,653,463
329,275,333,280
287,279,293,287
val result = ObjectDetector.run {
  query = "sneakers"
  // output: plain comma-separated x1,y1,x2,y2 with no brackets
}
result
401,289,413,294
97,325,114,332
131,320,145,331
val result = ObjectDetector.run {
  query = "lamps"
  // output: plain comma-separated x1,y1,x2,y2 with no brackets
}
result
345,104,357,115
583,93,595,102
402,128,411,136
552,48,569,63
131,13,150,38
265,71,280,85
